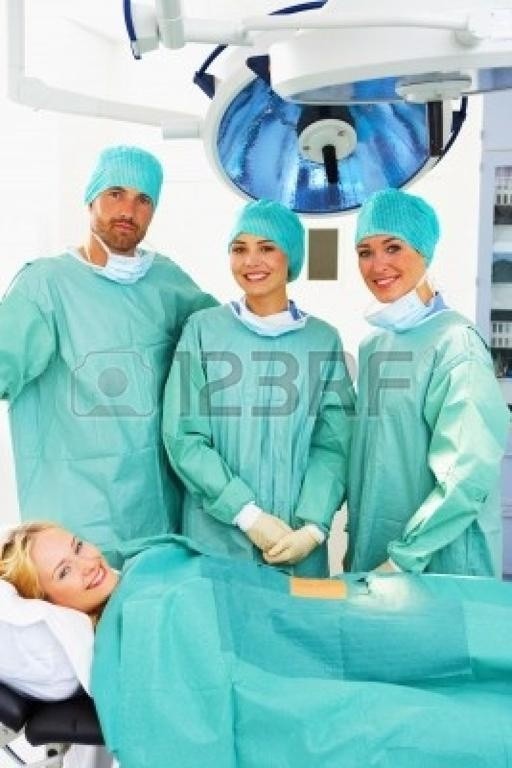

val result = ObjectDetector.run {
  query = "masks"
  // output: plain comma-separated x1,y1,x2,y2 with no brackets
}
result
359,288,434,335
236,302,307,340
93,245,159,288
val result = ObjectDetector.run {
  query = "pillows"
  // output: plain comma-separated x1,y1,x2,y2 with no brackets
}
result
0,579,96,702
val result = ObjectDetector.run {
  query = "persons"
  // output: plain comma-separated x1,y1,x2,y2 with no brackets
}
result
0,521,121,632
0,144,224,570
162,197,358,582
343,187,509,579
